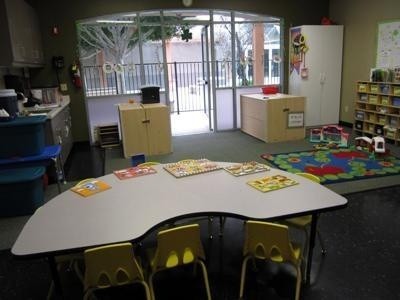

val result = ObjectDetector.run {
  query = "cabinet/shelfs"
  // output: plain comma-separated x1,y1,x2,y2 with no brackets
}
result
352,80,400,146
287,25,343,128
98,123,120,148
44,102,75,184
0,0,47,69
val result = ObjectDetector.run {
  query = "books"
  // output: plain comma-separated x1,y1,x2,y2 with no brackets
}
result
162,159,223,179
113,164,157,180
222,161,270,177
70,178,112,198
246,173,300,194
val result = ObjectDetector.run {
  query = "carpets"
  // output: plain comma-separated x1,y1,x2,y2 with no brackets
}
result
260,146,400,195
63,140,105,181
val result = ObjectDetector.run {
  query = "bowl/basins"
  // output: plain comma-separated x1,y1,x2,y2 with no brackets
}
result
262,87,278,94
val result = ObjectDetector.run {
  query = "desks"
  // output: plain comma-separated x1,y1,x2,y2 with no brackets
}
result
11,162,348,300
118,102,172,159
0,145,65,194
239,91,305,144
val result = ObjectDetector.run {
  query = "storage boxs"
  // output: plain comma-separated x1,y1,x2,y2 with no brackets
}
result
1,165,46,218
0,115,48,156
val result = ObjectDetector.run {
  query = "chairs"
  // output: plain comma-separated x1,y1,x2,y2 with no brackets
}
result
239,219,301,300
147,224,211,300
170,159,213,240
83,243,151,300
282,173,320,282
